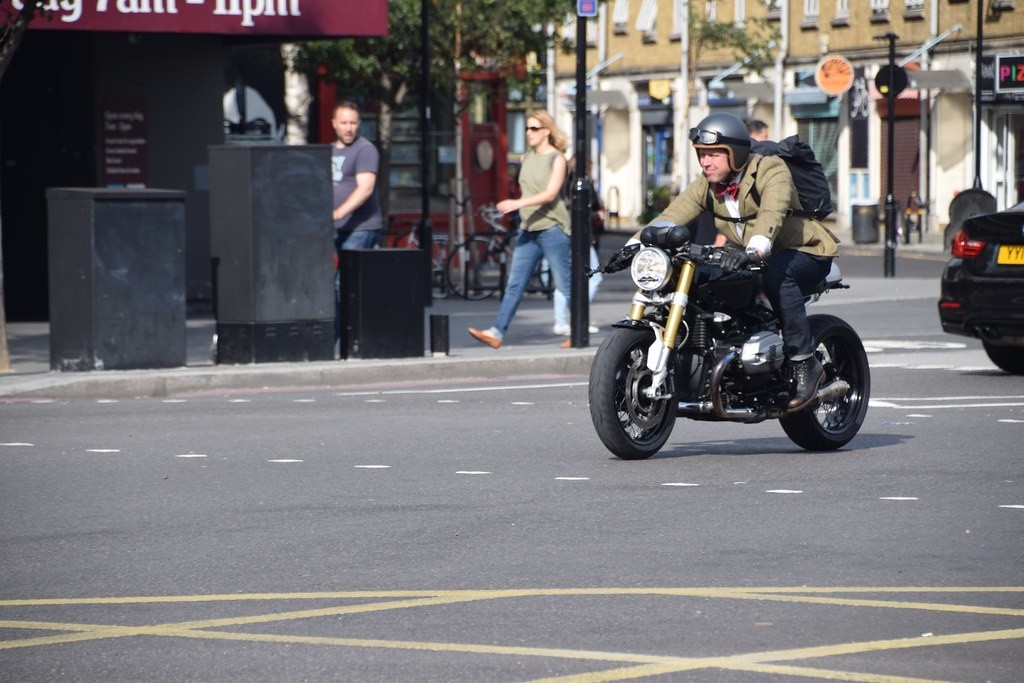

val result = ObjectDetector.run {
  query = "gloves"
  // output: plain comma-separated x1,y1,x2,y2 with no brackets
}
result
601,249,637,273
720,251,759,272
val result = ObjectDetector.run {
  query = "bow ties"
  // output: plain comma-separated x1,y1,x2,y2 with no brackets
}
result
714,182,740,200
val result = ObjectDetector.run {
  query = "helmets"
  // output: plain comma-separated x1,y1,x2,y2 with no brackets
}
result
691,112,750,172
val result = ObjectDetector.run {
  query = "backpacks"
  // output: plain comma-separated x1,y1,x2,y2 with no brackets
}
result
706,135,833,221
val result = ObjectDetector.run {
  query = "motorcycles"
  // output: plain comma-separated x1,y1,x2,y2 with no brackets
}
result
583,226,872,459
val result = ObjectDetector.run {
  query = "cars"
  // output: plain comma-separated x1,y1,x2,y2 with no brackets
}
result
938,198,1024,376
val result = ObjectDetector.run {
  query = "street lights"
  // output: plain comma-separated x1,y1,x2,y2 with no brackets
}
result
872,31,899,277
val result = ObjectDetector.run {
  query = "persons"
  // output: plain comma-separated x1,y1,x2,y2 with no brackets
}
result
742,119,769,140
626,111,826,411
468,108,602,348
330,101,384,345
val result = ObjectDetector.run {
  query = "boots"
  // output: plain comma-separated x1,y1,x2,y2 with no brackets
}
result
786,354,826,413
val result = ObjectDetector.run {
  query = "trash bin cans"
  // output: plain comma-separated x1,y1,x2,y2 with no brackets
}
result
47,189,187,370
852,204,879,244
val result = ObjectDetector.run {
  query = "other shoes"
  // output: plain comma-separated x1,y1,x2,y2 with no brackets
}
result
560,338,571,347
468,327,502,349
553,324,571,335
589,326,599,333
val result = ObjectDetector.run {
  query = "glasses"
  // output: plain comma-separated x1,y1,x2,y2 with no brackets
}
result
689,128,726,145
525,126,545,133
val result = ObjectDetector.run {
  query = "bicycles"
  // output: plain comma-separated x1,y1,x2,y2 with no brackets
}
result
330,208,557,302
